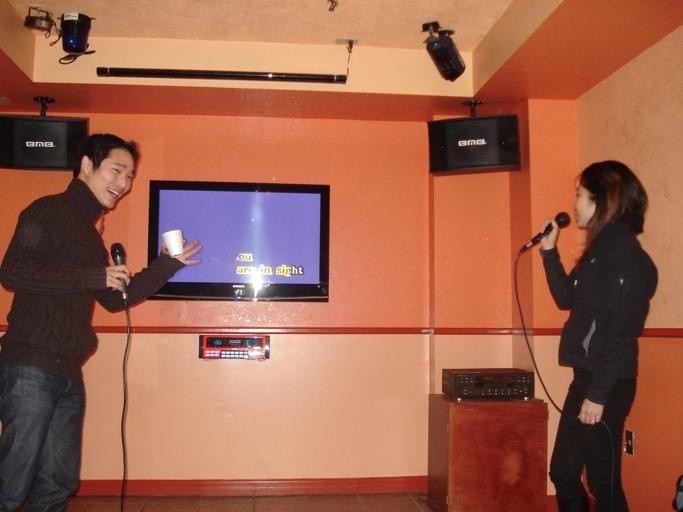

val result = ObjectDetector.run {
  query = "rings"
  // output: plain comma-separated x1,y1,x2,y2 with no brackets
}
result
590,420,594,424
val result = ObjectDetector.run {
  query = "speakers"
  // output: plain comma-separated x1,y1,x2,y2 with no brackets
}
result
0,114,91,172
427,113,522,175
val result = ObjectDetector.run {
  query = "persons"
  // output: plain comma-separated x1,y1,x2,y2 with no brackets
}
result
0,132,203,511
539,159,659,512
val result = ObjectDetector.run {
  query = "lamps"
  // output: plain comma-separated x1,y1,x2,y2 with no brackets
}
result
417,20,468,83
25,4,97,66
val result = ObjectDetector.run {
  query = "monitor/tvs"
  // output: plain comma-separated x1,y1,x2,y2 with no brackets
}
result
147,179,332,301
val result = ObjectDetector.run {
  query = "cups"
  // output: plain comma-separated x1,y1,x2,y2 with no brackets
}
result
161,229,184,259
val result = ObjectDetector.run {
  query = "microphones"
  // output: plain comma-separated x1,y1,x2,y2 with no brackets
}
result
109,241,129,304
519,211,571,253
